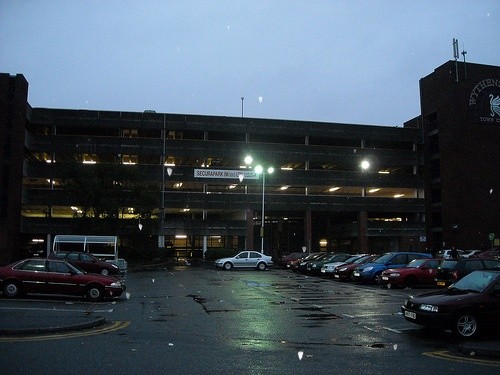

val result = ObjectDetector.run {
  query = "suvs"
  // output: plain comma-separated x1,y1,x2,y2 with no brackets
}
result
44,250,120,277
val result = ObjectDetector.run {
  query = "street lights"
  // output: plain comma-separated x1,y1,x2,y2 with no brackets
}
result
253,163,274,254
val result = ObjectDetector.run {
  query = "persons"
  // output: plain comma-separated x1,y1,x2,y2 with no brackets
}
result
449,247,460,259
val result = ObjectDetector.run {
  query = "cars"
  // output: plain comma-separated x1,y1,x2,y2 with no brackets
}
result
398,269,500,341
429,257,500,290
214,250,274,271
0,256,127,302
276,248,500,290
